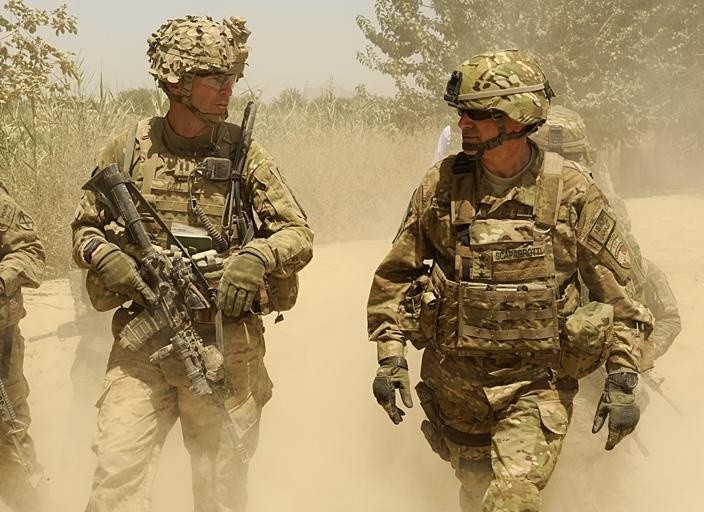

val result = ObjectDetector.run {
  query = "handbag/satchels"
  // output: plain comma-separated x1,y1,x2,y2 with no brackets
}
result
265,273,299,315
559,301,613,380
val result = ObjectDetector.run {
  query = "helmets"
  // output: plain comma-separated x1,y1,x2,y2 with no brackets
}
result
527,105,588,153
444,49,556,126
149,15,251,82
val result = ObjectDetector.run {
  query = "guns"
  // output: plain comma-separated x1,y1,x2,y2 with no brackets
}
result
587,366,684,457
0,378,30,475
28,307,121,342
80,162,251,464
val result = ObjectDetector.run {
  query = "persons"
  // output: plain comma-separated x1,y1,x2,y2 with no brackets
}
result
434,119,462,163
70,262,122,401
1,183,48,510
71,16,312,512
368,50,654,512
567,154,680,459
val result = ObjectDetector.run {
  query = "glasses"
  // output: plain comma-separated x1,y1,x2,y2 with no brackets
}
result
457,109,491,120
202,75,239,90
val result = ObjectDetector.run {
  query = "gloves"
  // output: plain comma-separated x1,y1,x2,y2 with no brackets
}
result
216,252,266,317
91,243,157,308
373,356,413,425
591,378,641,451
